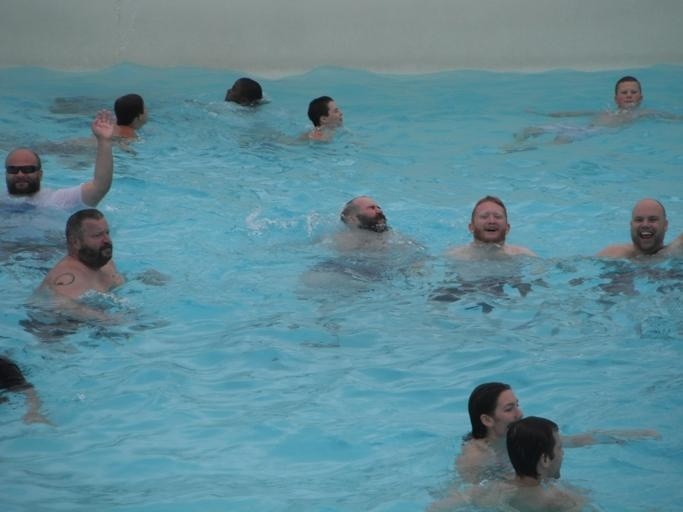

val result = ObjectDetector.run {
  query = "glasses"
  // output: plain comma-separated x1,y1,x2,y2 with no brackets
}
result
4,164,40,174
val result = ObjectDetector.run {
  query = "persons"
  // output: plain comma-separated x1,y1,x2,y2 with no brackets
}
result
472,413,598,512
586,195,681,266
424,380,666,500
497,76,681,154
11,92,147,158
438,193,547,272
21,205,171,344
303,92,345,143
0,352,49,425
221,74,268,109
295,195,425,297
3,108,116,214
